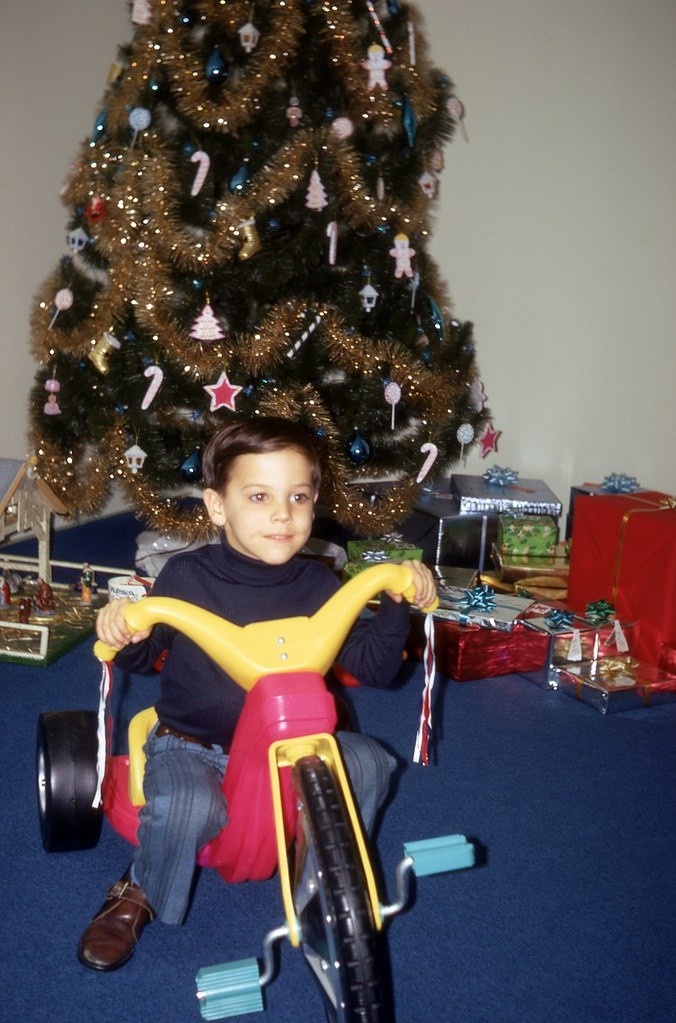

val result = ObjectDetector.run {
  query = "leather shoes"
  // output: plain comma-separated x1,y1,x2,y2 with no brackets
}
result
78,860,154,972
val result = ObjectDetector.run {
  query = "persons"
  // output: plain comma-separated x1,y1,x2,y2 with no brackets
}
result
78,417,438,973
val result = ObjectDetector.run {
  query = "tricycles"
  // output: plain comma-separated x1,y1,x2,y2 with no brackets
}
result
36,558,476,1023
